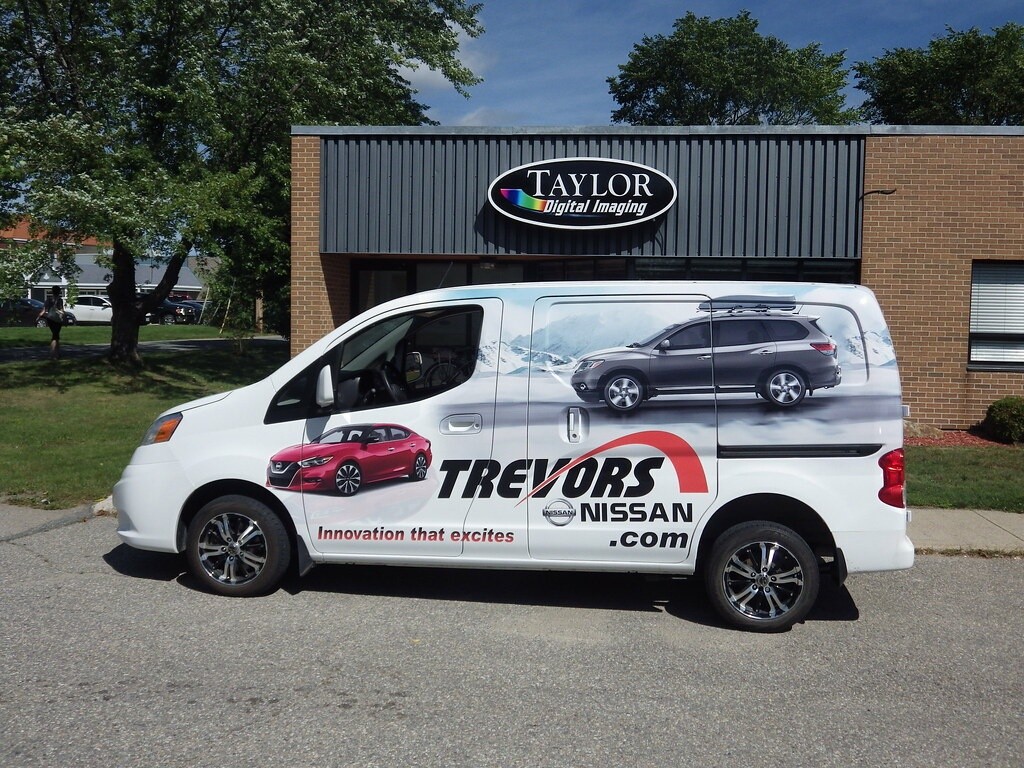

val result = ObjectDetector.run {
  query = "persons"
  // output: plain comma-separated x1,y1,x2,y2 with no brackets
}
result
36,285,68,360
433,345,478,389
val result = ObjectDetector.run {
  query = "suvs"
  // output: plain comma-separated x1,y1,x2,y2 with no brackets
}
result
569,292,843,416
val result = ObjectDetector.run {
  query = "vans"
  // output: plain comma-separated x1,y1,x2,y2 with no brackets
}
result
112,282,914,633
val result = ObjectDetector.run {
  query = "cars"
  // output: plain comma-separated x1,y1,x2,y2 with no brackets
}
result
0,298,64,328
265,421,432,496
134,291,212,326
61,295,114,326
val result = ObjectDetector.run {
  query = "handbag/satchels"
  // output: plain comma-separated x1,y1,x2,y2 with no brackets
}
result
47,298,64,322
